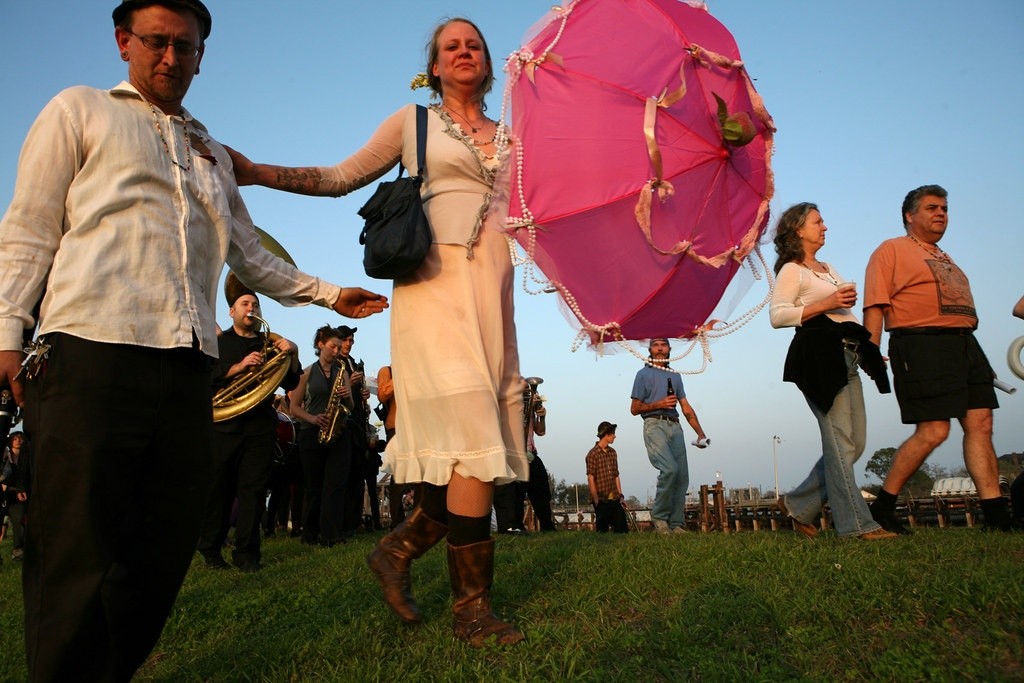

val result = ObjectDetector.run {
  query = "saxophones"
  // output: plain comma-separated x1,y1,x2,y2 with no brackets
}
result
317,359,345,445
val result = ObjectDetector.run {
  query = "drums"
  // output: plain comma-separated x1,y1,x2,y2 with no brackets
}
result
273,410,296,465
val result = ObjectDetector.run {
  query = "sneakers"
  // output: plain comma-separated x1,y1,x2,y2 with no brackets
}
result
778,497,817,538
861,529,897,541
673,526,687,534
653,520,673,536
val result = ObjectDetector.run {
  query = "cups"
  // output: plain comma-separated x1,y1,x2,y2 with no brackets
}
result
837,282,856,306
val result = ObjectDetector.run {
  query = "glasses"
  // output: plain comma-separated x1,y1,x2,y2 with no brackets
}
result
125,30,202,61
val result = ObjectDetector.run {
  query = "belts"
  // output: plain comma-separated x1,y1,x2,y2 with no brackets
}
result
841,342,859,353
645,414,679,422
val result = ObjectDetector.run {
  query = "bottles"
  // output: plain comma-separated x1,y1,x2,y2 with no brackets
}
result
667,378,676,408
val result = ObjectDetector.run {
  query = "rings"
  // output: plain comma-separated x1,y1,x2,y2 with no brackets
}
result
362,307,365,312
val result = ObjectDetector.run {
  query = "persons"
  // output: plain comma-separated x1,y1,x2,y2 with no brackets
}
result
440,102,488,133
628,336,711,534
202,15,539,649
857,185,1024,532
494,371,560,532
583,422,629,535
0,0,392,682
764,197,902,539
0,262,410,573
1012,294,1024,318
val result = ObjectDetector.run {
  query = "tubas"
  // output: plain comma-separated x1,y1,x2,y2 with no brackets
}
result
524,377,543,453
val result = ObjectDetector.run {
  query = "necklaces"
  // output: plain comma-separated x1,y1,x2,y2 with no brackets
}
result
472,120,500,145
799,257,838,285
906,233,952,272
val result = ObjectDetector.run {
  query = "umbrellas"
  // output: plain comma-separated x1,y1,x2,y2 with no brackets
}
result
482,0,780,373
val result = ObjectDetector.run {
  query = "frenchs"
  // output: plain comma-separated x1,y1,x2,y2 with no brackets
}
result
212,224,300,425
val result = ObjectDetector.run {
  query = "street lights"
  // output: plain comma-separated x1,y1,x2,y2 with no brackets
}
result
747,483,751,501
772,435,782,500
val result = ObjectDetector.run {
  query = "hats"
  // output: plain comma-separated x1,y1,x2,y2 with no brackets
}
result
337,326,357,339
597,422,617,437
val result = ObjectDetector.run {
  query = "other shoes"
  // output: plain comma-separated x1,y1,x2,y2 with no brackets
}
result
289,528,302,539
278,524,288,533
264,528,277,539
980,497,1020,532
869,487,915,536
230,553,261,571
205,554,231,571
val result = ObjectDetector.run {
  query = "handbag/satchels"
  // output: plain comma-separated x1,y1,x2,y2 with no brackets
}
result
374,402,388,421
358,105,432,280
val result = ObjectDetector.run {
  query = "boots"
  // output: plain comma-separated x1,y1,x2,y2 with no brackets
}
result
366,504,450,624
446,536,525,650
